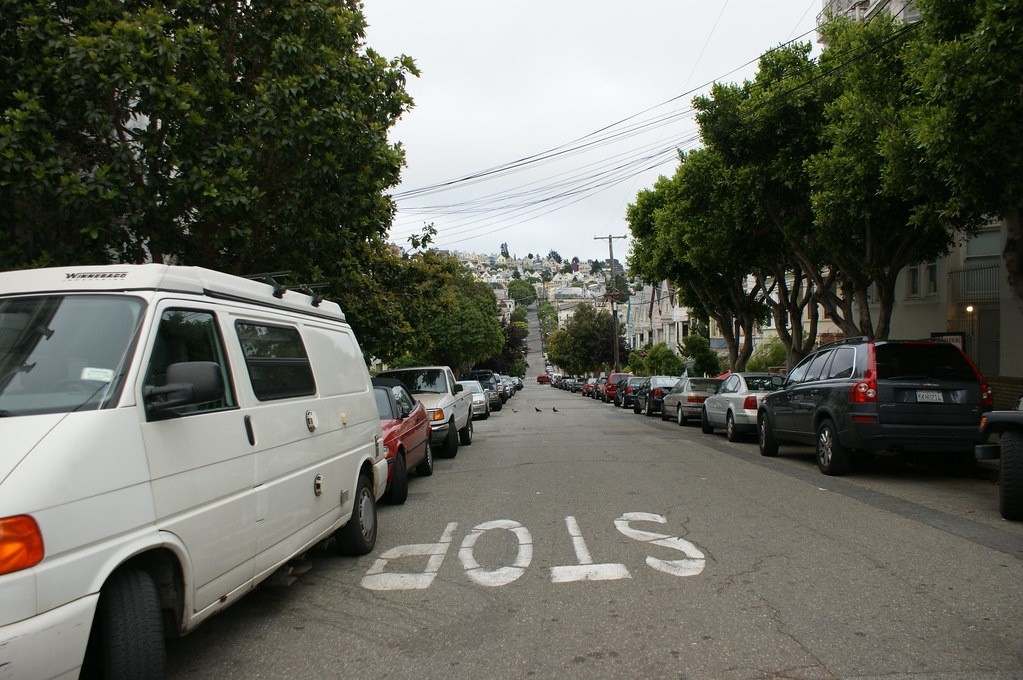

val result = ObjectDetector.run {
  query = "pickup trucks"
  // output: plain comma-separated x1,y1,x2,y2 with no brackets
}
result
370,367,474,458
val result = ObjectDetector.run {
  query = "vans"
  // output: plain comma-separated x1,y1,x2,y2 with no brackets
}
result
551,374,562,387
0,264,391,680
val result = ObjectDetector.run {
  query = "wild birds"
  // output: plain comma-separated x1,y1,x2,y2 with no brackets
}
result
512,408,518,413
534,407,542,412
552,406,559,413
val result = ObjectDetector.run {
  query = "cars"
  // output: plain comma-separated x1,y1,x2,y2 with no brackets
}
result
453,380,491,419
613,376,650,409
367,377,436,506
593,377,608,401
542,352,555,376
660,376,725,427
633,376,682,417
557,378,587,393
974,397,1023,520
701,372,788,442
494,373,524,404
582,378,598,397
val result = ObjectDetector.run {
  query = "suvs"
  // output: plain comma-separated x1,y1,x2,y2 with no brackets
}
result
757,337,995,478
603,373,634,402
464,369,503,412
537,375,552,384
